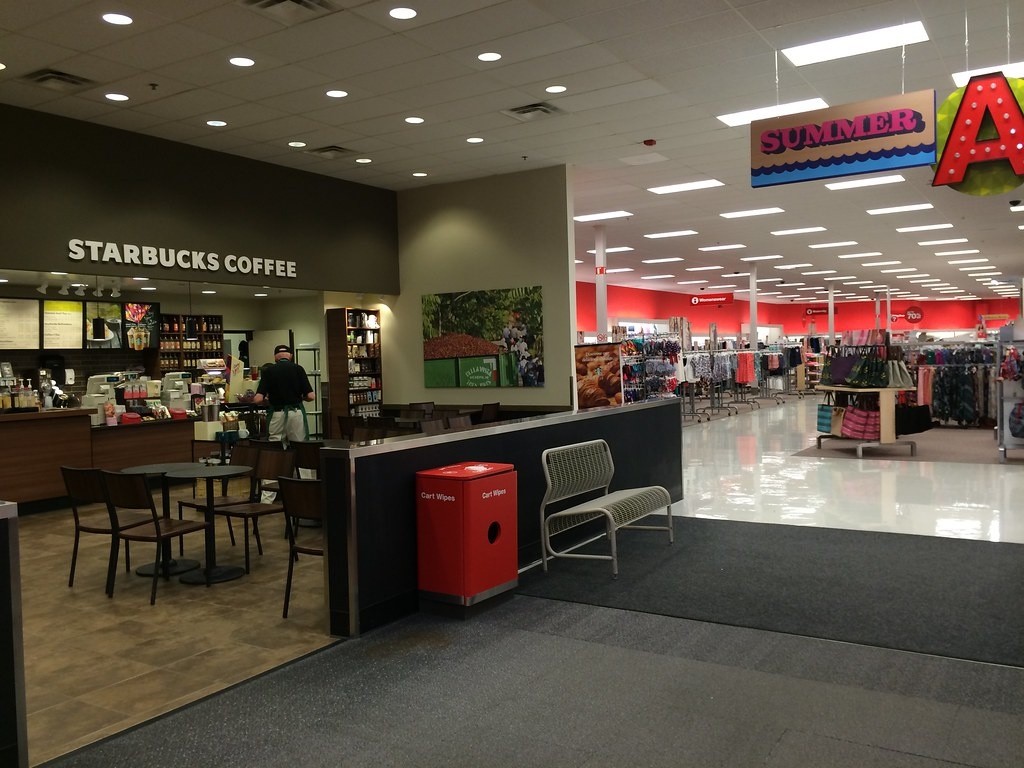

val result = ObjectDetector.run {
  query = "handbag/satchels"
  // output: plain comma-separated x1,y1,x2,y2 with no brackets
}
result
819,343,914,389
817,391,933,442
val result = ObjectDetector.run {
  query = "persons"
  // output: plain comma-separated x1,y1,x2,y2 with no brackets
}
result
502,319,545,387
255,344,316,505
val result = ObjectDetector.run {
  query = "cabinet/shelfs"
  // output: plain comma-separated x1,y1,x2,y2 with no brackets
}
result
144,313,224,383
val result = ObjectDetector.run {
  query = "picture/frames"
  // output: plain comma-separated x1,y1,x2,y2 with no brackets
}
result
123,301,160,351
84,300,123,351
0,297,41,351
41,298,84,351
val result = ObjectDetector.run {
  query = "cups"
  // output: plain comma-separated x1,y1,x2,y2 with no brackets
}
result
238,428,250,438
199,404,209,422
250,365,259,380
127,331,150,350
210,404,219,422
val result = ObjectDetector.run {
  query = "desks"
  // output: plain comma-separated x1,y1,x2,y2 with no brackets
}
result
164,466,252,585
305,439,350,449
326,307,384,422
120,462,207,577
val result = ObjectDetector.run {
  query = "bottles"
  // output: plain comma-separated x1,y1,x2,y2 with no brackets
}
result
202,335,221,351
350,390,367,403
160,354,179,370
159,336,180,349
202,353,221,359
349,376,377,389
184,355,200,368
182,316,199,331
200,316,222,331
182,336,201,351
349,330,355,342
160,318,179,333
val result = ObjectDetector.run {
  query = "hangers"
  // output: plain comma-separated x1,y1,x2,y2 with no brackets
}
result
621,331,803,398
919,342,990,355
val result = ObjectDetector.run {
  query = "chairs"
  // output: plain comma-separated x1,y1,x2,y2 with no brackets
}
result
61,402,502,619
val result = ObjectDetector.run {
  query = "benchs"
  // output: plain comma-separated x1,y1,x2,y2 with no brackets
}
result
539,439,674,580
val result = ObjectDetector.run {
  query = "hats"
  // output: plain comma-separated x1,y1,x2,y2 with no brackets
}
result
274,345,292,363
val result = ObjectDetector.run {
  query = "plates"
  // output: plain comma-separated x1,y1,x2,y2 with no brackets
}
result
86,328,114,342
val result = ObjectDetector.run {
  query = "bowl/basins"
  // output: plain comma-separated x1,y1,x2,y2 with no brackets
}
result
107,318,122,327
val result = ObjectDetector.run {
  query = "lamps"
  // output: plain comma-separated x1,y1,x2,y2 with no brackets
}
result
36,273,122,298
